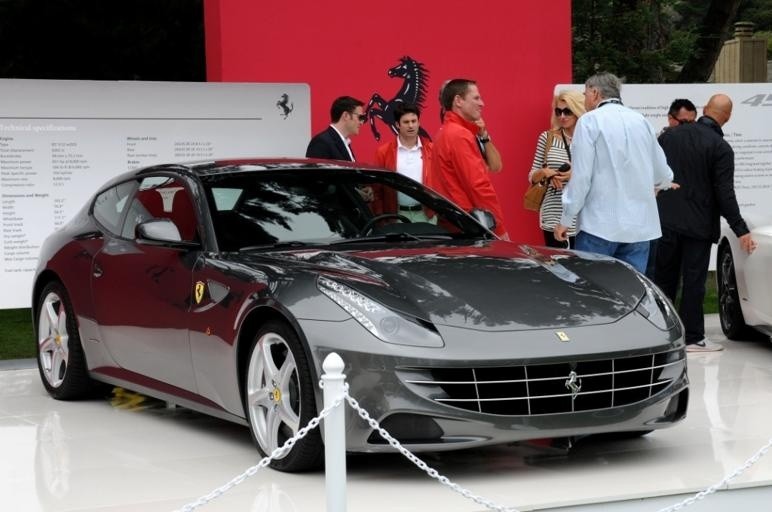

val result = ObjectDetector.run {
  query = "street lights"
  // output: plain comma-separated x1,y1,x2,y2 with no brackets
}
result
523,162,548,210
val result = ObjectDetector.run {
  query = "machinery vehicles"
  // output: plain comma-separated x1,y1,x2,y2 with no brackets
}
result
33,155,690,475
717,222,772,339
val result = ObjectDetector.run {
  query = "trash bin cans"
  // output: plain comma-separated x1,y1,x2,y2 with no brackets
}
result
478,135,492,142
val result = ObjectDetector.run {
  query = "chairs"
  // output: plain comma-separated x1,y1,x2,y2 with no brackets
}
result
686,338,723,352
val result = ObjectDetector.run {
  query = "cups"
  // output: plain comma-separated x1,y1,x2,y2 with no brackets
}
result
555,107,572,116
672,116,693,125
353,113,368,122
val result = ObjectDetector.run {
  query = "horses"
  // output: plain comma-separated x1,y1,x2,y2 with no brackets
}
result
559,163,571,173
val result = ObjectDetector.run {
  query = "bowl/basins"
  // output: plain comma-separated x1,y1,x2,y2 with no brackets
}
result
561,128,574,141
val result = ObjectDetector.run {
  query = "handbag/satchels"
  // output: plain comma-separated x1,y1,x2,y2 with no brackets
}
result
546,173,558,184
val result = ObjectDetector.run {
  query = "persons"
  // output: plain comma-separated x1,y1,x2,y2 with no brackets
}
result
657,95,756,353
653,99,699,141
438,80,505,173
527,87,591,249
302,96,371,207
431,78,513,242
552,71,680,274
371,104,440,227
